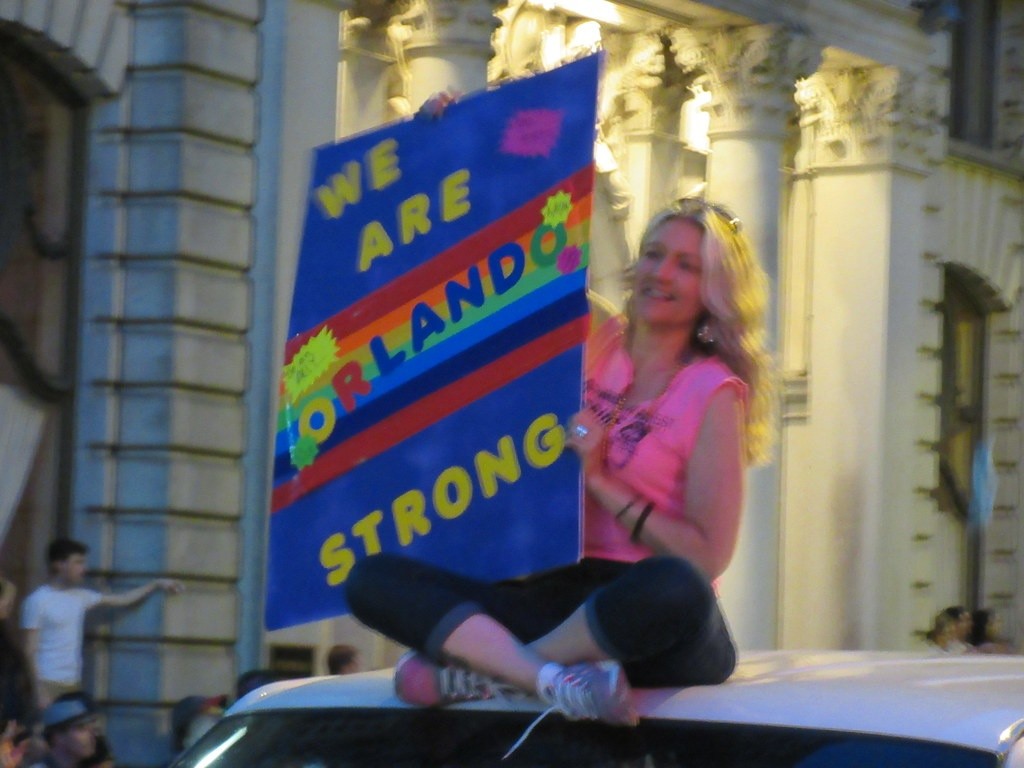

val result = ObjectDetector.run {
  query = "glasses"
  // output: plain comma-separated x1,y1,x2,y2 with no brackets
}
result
677,196,738,234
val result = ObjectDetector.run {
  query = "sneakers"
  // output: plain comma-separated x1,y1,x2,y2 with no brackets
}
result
394,641,530,709
501,654,640,761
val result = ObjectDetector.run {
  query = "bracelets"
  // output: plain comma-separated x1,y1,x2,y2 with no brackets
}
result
631,502,656,543
615,494,643,520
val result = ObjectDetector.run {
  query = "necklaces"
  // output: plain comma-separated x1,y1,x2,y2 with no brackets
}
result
601,366,677,469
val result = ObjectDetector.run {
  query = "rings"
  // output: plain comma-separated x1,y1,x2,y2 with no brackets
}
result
574,424,589,438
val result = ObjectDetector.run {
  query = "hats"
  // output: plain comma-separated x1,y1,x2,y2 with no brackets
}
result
40,699,99,742
169,693,228,751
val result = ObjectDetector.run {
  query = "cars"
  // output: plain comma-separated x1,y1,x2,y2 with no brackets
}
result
170,649,1024,767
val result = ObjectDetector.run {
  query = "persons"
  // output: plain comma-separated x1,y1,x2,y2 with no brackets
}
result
0,537,361,768
925,605,1014,654
344,87,776,758
